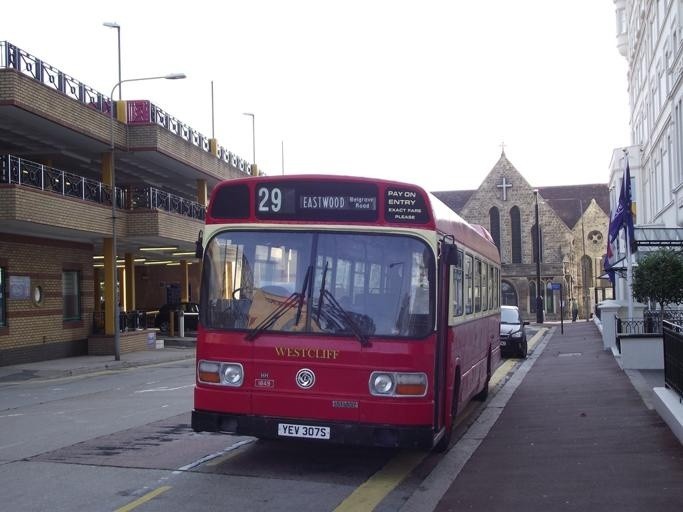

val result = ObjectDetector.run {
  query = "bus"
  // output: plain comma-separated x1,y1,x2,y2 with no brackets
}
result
191,174,500,456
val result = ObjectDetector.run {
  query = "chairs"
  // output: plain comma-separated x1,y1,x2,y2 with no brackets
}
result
252,285,290,305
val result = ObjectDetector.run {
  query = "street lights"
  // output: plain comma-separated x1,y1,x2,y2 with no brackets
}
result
241,112,256,166
103,22,121,100
110,72,186,361
533,188,543,324
577,198,590,322
387,260,404,325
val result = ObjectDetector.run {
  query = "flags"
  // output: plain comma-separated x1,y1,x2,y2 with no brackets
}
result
610,161,635,243
604,212,615,284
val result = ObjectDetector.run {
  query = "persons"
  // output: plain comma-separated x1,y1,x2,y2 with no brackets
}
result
573,309,578,321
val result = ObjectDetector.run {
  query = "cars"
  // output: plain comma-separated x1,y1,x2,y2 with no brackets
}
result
501,304,531,359
